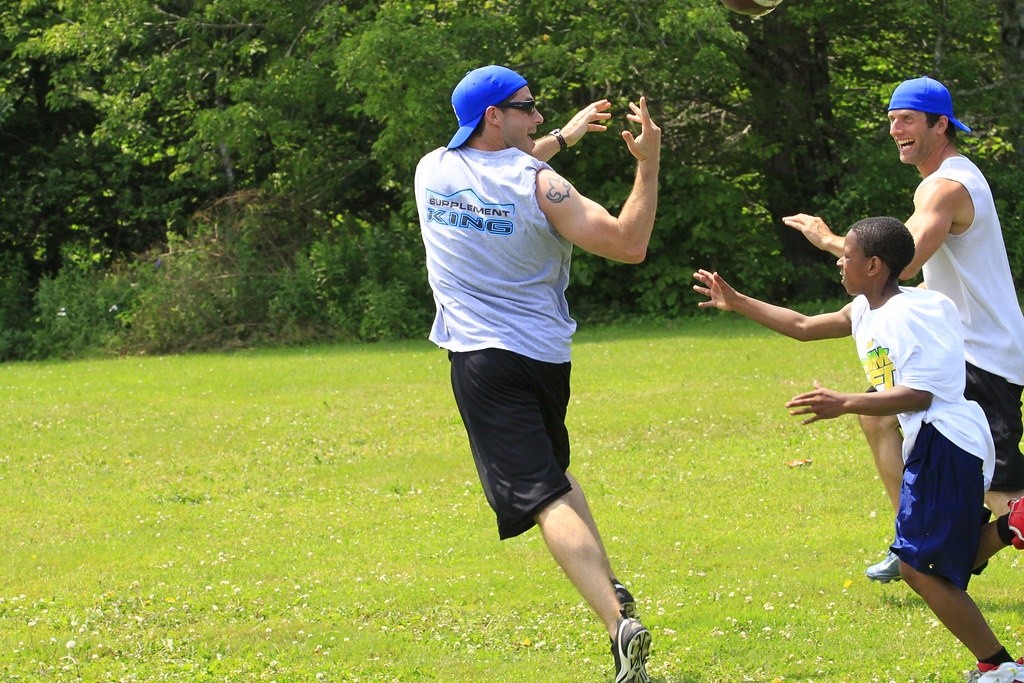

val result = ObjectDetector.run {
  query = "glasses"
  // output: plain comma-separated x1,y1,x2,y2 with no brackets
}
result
496,100,536,114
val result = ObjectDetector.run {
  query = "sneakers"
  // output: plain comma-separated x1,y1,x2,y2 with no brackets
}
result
865,550,901,583
610,609,651,683
966,659,1023,683
611,578,637,617
1008,496,1024,550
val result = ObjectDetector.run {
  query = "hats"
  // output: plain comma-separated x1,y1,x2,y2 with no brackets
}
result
887,76,972,134
447,64,527,150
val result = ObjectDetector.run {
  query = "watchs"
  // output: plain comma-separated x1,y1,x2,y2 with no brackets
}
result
547,127,569,153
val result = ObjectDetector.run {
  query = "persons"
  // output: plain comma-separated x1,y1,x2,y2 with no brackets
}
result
413,63,661,683
781,76,1024,586
692,215,1024,683
